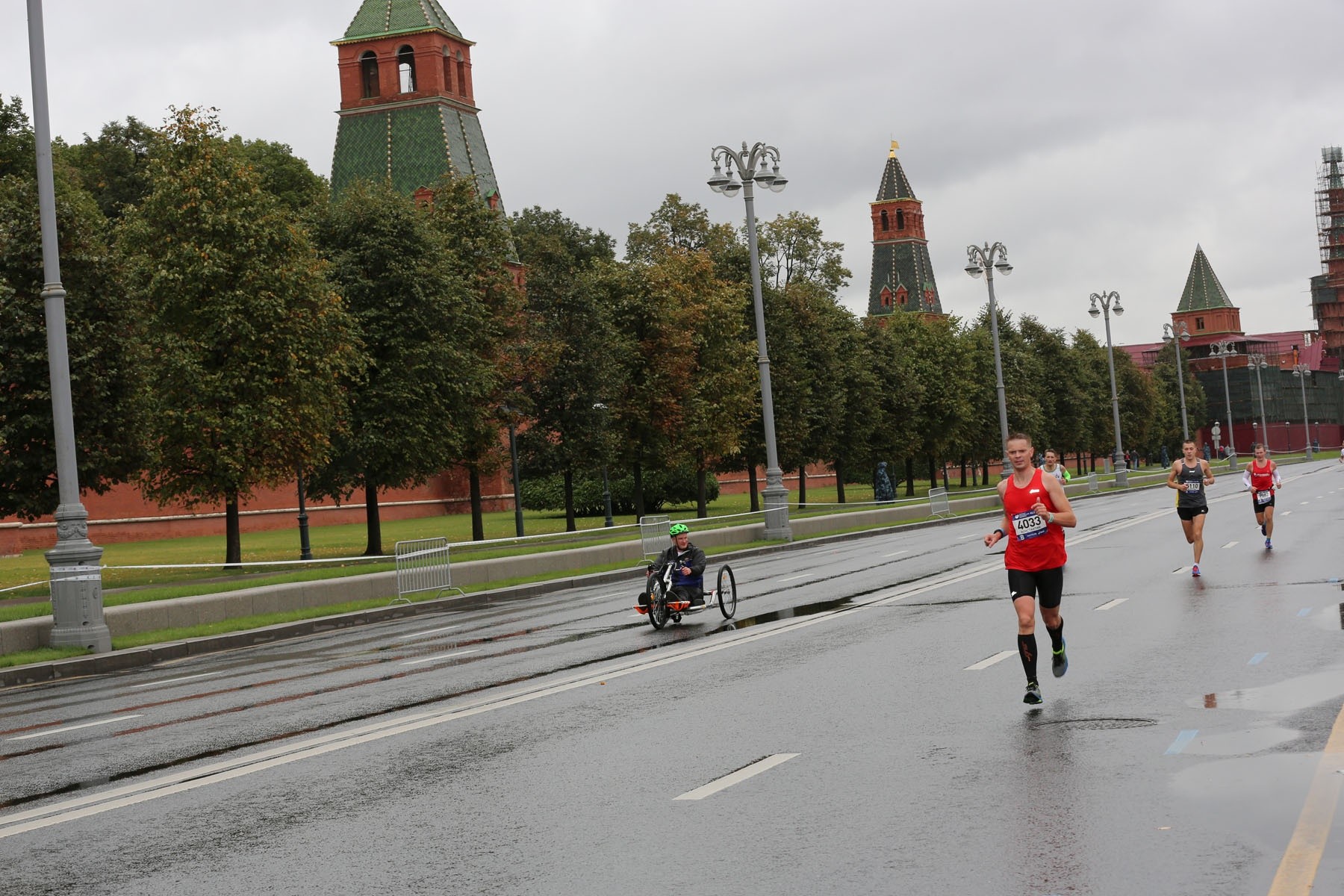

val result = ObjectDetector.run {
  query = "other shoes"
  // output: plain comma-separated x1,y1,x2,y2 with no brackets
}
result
1264,540,1272,549
1261,520,1267,536
638,592,648,615
666,590,682,609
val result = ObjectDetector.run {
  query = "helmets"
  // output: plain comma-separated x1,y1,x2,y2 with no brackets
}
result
669,523,689,537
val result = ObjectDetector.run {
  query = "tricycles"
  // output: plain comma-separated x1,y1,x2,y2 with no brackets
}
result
632,560,737,630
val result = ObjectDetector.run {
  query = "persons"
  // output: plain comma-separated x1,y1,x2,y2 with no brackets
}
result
638,524,707,611
1126,439,1344,579
984,433,1076,704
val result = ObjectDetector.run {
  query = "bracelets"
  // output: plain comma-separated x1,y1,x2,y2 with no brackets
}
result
1047,512,1054,524
995,529,1004,539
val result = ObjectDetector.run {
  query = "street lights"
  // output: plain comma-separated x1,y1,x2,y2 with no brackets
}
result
1214,421,1220,460
1292,362,1314,460
1315,422,1320,444
1247,352,1270,458
1160,320,1192,440
1208,339,1237,470
707,141,795,541
1285,422,1292,454
1088,289,1128,486
1252,422,1258,444
964,242,1017,481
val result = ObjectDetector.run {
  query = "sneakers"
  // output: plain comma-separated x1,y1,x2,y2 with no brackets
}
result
1192,565,1201,577
1023,680,1043,705
1051,638,1068,678
